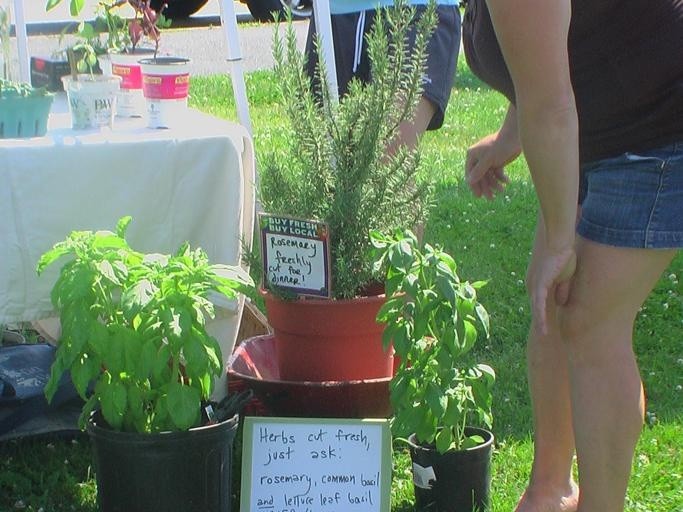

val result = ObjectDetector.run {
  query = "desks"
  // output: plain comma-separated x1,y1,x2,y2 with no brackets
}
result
0,98,257,402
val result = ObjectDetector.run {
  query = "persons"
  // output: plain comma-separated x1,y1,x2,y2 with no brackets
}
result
462,1,683,512
301,1,461,243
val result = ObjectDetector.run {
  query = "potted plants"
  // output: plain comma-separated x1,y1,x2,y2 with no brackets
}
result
0,0,193,132
253,0,440,382
371,228,495,512
35,215,262,512
